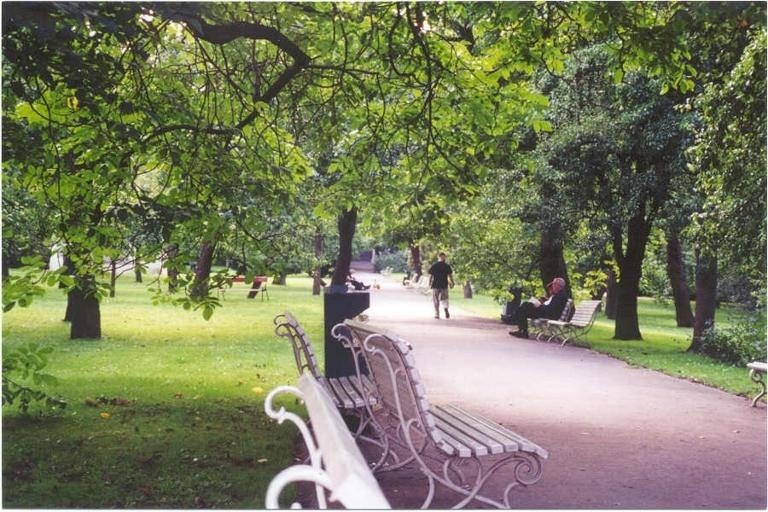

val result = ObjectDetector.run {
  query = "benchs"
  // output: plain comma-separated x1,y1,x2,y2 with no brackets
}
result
402,271,419,285
412,274,432,296
264,371,392,509
521,297,573,341
546,300,603,350
746,360,767,408
330,319,550,510
273,310,400,467
217,275,269,301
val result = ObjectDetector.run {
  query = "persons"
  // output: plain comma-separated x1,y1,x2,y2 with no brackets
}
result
501,278,567,337
501,282,554,334
347,270,371,290
428,252,455,318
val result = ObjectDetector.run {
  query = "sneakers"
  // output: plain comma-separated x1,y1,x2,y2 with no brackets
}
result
434,315,440,319
509,328,529,339
500,313,520,324
445,308,450,319
